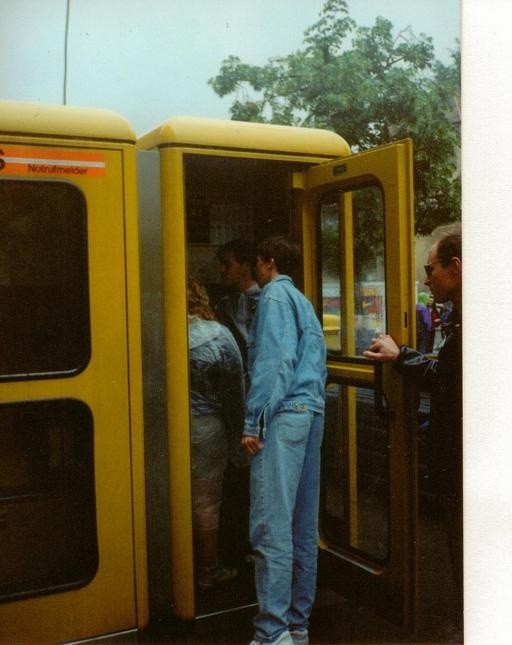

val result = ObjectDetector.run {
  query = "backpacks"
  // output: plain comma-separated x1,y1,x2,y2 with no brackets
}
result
440,311,455,335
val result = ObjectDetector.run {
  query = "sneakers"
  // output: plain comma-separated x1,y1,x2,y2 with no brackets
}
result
198,567,238,590
245,554,256,563
248,630,309,645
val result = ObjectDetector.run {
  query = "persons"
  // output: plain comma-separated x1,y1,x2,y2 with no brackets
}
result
241,230,326,645
322,287,462,358
208,242,264,577
361,230,462,644
185,264,250,595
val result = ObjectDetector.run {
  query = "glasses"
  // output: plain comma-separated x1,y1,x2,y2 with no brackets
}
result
424,258,452,277
